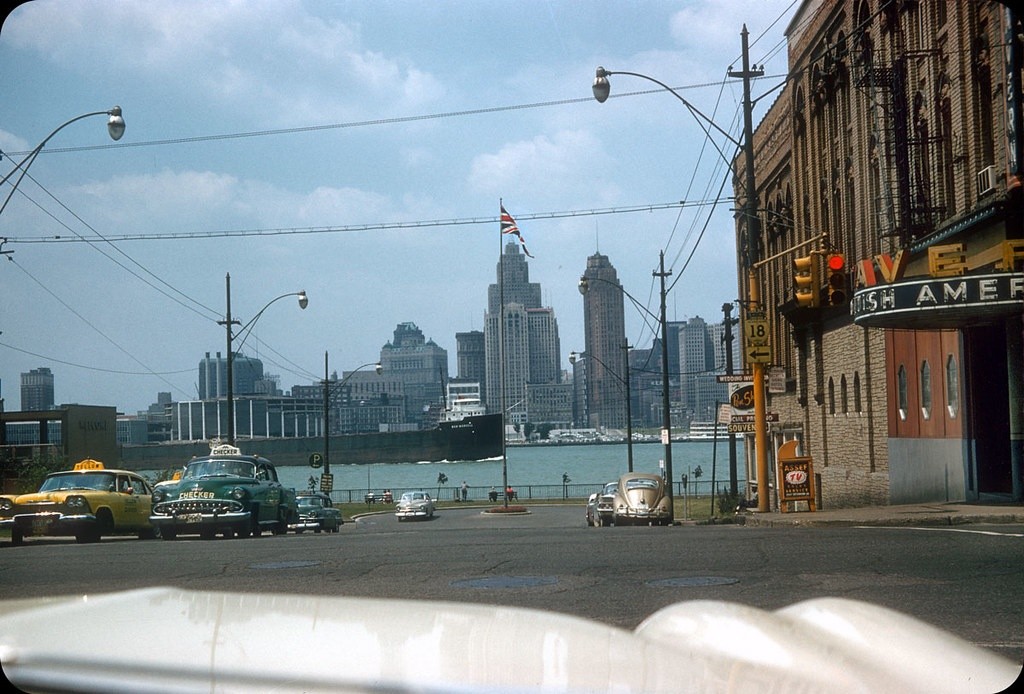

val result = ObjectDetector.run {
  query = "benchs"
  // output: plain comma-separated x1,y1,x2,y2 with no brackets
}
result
489,491,519,502
364,494,394,504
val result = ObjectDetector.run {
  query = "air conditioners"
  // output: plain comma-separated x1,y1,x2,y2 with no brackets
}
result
976,164,998,196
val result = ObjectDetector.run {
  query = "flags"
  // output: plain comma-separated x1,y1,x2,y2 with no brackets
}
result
501,205,534,257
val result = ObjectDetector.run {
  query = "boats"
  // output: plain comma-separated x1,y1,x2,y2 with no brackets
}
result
687,421,744,442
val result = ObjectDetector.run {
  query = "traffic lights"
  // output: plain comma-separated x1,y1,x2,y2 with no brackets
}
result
826,254,847,305
793,253,819,308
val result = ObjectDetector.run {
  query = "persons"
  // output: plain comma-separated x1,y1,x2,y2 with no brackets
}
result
114,478,133,493
461,481,469,501
382,489,393,504
507,485,514,502
368,492,375,504
237,464,258,479
489,487,498,502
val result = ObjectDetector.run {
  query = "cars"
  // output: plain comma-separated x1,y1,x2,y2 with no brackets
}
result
586,472,670,525
0,456,161,544
149,444,344,540
395,491,433,521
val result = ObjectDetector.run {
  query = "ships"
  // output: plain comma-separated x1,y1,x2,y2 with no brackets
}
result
118,364,498,471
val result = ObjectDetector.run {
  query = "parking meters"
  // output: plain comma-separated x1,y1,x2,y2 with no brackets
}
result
681,474,687,521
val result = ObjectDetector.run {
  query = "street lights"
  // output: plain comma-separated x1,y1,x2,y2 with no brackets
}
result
594,65,771,513
324,363,383,507
568,351,634,473
578,275,674,523
227,291,309,443
